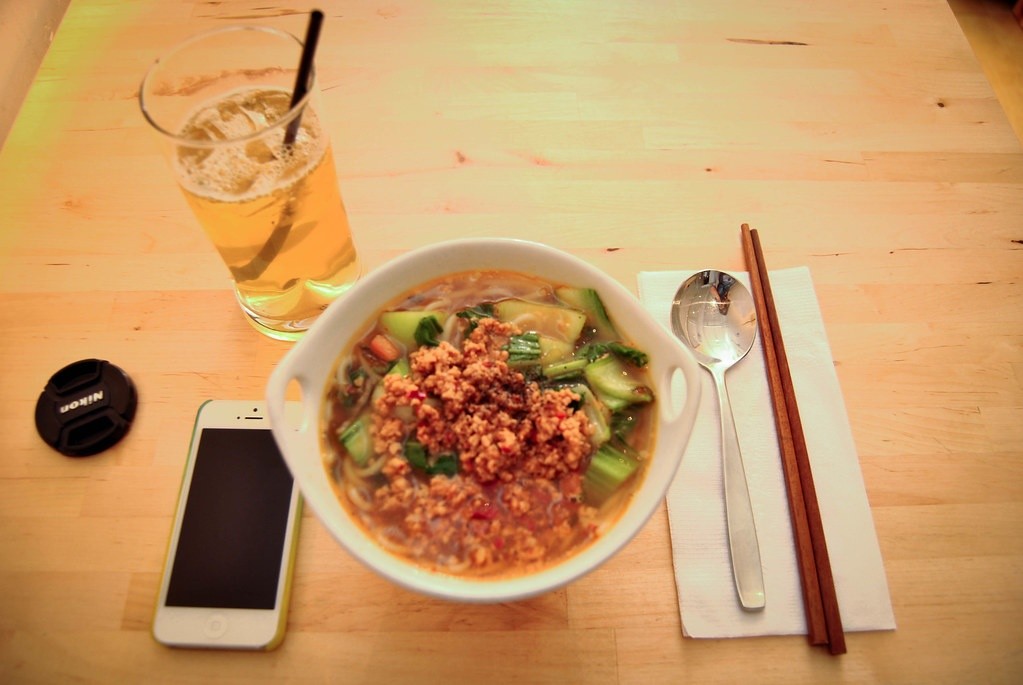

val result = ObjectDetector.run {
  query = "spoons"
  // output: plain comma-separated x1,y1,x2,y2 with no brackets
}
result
669,270,769,612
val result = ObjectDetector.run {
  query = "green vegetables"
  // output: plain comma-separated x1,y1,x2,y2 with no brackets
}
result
338,286,654,504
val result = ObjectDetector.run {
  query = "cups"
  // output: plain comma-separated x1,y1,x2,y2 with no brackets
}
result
139,25,368,343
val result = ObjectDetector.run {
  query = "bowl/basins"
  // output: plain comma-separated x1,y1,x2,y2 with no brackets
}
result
263,236,704,603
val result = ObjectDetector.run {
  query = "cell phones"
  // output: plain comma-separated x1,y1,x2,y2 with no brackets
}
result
152,400,303,652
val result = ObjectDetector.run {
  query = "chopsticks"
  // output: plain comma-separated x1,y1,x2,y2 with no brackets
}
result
741,221,848,659
